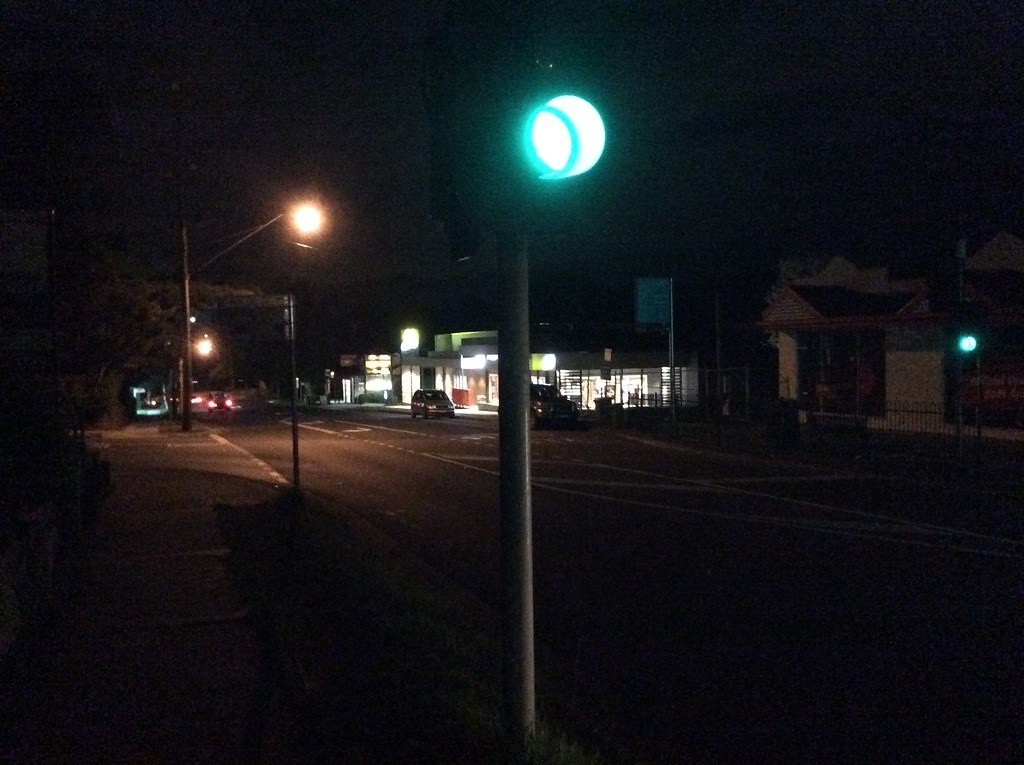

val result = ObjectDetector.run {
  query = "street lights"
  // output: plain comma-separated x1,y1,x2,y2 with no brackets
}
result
283,198,325,491
489,74,607,750
958,335,982,443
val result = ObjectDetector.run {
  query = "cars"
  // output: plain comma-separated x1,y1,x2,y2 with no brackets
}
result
410,388,454,419
191,392,233,413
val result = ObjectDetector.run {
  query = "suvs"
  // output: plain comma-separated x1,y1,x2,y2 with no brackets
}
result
530,382,579,432
